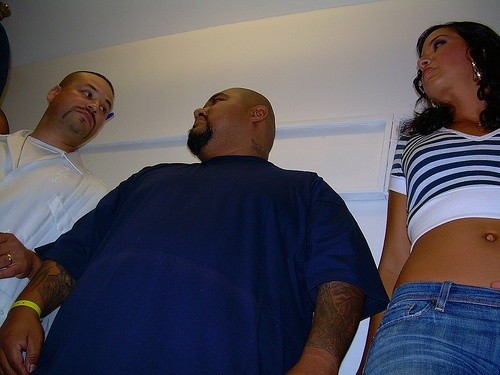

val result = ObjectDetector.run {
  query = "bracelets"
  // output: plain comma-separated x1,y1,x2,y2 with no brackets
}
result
6,299,43,318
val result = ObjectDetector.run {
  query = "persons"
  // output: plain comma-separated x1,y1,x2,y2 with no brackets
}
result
0,86,389,375
356,21,500,375
0,69,117,375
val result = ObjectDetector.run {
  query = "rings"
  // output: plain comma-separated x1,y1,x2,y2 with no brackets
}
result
6,253,13,265
